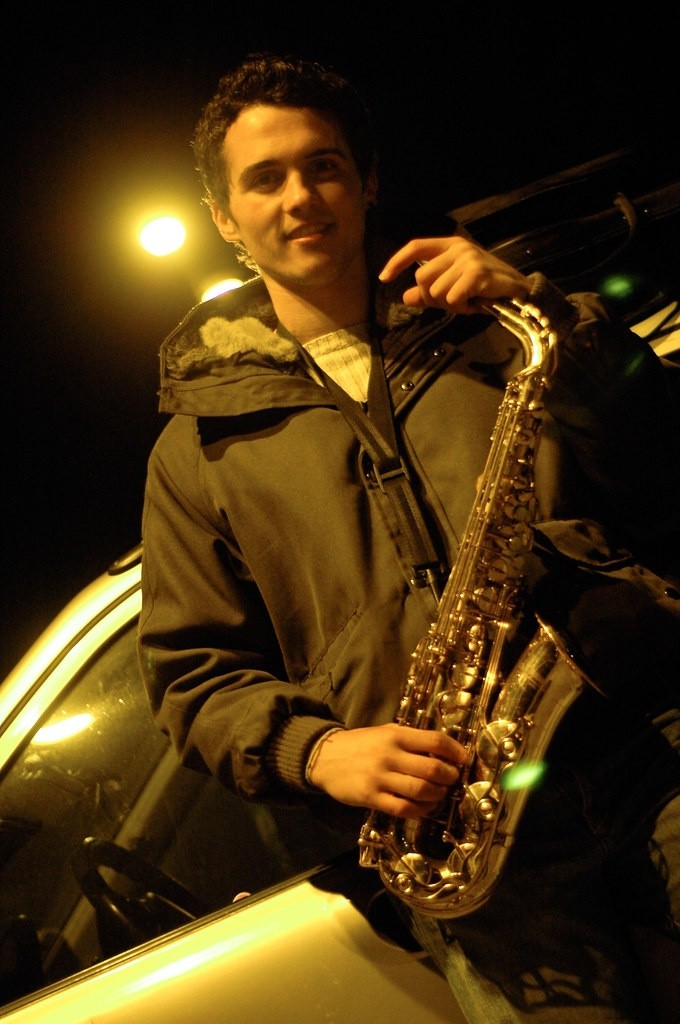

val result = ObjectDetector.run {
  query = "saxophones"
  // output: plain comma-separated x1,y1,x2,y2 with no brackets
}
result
356,263,606,917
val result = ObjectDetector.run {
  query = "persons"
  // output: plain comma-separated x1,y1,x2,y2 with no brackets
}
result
135,53,680,1024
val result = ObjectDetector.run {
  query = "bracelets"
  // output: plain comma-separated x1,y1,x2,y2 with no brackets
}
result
305,728,344,785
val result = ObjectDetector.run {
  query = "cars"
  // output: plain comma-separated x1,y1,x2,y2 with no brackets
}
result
1,144,678,1022
